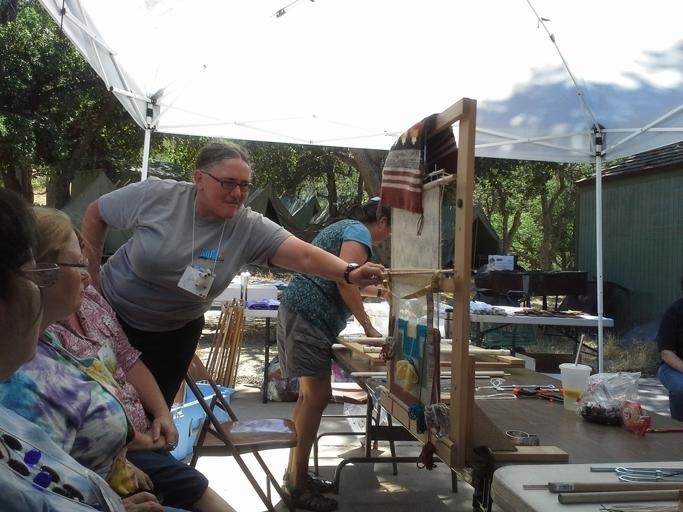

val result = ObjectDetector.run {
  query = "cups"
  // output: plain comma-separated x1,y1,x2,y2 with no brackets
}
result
559,361,591,413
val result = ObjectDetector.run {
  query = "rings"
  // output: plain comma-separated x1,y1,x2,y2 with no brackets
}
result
169,442,176,448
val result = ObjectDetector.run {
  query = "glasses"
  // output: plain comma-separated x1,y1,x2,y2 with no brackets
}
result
201,168,254,194
6,259,61,290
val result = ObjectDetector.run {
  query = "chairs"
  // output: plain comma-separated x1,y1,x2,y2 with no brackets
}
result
314,322,399,476
184,354,299,512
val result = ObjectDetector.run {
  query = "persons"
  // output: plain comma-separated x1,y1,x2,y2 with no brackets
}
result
277,193,393,512
0,183,185,511
44,233,236,511
656,297,683,422
1,208,157,495
80,139,388,425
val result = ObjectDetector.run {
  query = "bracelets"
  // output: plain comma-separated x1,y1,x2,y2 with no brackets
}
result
344,262,360,285
377,283,383,298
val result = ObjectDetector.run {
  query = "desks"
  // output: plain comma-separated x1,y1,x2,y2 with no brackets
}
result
438,302,615,374
244,309,354,405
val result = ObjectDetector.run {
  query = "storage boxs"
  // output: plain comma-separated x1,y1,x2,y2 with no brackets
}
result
170,383,234,461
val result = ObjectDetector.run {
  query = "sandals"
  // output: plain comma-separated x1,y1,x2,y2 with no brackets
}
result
282,483,338,512
283,467,337,493
